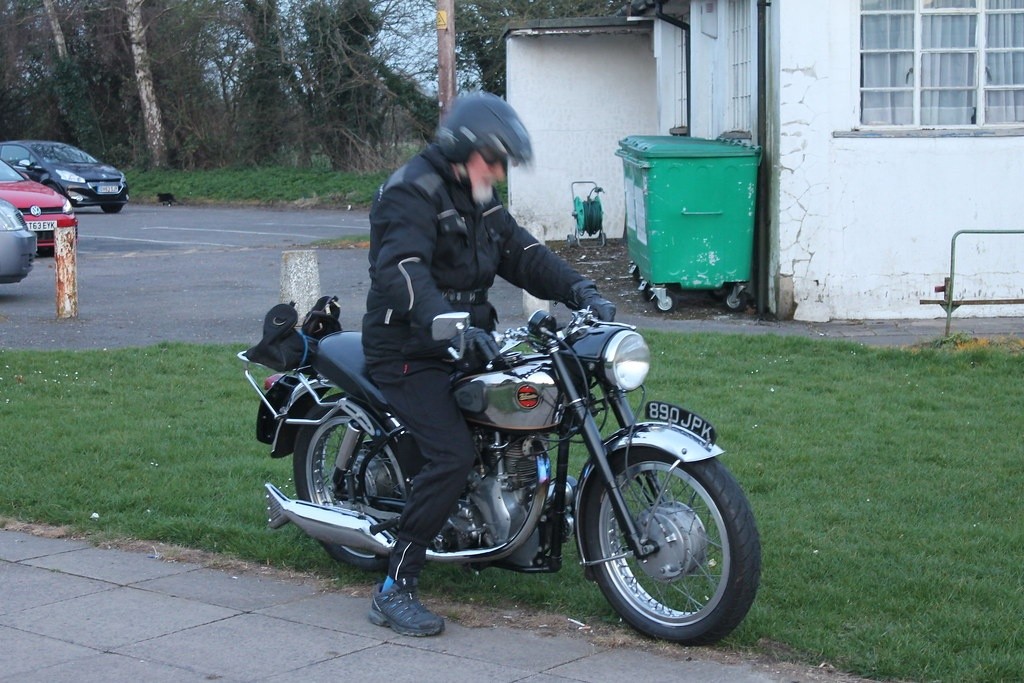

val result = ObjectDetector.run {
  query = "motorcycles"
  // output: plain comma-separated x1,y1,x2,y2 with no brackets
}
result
234,294,763,645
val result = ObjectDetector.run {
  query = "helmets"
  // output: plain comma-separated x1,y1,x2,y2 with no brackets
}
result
436,91,534,160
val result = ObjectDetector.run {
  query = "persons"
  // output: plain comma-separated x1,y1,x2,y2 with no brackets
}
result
362,91,617,635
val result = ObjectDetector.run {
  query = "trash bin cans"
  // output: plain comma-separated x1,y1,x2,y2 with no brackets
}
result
615,133,764,313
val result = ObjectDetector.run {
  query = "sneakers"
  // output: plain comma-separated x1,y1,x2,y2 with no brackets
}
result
369,581,444,637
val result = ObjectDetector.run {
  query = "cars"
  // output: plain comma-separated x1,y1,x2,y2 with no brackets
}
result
0,139,130,214
0,159,78,258
0,198,38,284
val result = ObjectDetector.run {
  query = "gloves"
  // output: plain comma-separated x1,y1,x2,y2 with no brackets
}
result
450,327,503,362
578,293,616,327
